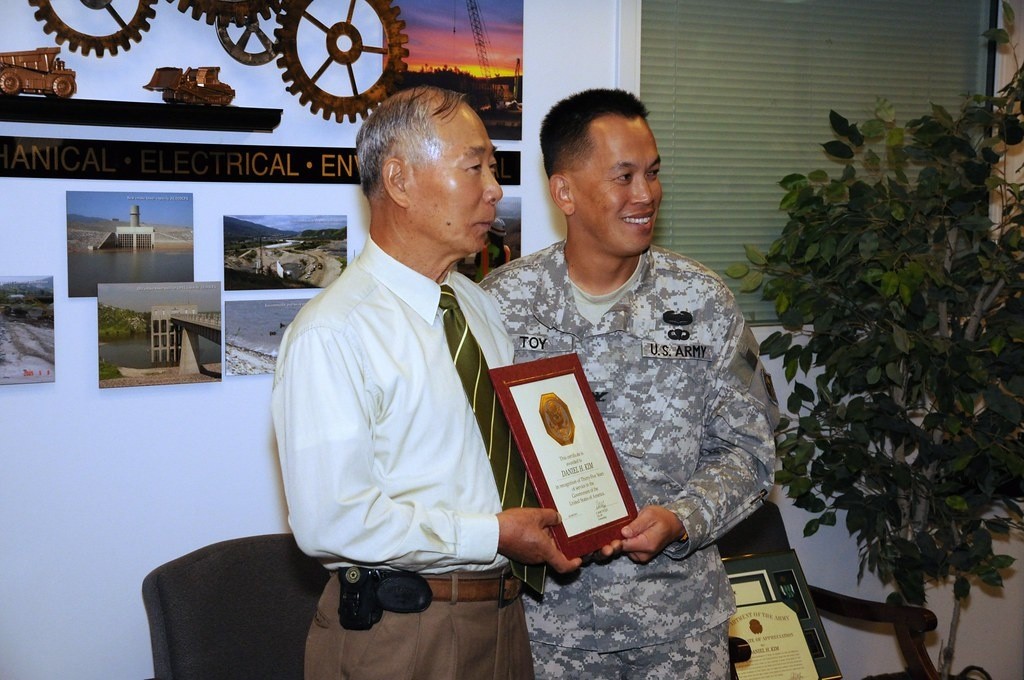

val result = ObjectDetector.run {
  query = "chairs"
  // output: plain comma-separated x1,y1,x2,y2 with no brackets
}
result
717,502,938,680
141,532,330,680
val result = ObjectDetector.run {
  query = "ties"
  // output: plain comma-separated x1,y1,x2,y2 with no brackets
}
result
439,284,546,597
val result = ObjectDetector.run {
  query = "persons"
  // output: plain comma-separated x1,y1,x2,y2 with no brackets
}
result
271,86,621,680
478,90,780,680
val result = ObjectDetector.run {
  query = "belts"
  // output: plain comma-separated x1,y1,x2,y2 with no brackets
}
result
427,565,525,609
